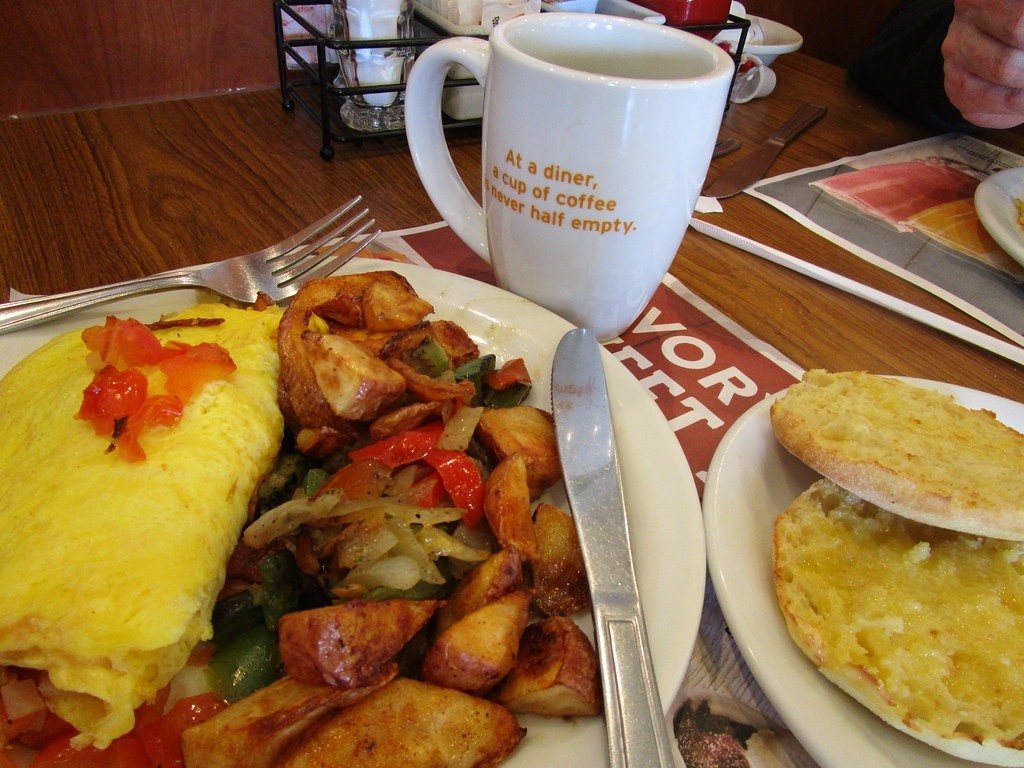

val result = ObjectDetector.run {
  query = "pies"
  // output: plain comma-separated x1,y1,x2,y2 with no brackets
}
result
0,297,285,748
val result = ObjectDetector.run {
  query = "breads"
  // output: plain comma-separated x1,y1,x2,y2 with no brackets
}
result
767,370,1024,542
770,478,1024,768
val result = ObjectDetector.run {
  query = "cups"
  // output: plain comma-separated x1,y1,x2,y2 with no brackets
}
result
402,11,736,345
728,54,777,103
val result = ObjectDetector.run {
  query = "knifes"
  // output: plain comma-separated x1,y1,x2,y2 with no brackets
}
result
550,327,677,768
700,100,827,198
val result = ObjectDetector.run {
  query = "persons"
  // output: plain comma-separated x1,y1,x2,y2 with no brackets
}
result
856,0,1024,135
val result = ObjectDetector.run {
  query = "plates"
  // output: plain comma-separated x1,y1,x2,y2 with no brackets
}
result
0,252,704,768
974,166,1024,268
702,373,1024,768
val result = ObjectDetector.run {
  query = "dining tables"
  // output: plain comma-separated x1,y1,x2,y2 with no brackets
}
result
0,51,1024,767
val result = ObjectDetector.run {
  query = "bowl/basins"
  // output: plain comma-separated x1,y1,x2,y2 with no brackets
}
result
711,13,803,66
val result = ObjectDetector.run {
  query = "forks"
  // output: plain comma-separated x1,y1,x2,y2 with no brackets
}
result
0,194,386,337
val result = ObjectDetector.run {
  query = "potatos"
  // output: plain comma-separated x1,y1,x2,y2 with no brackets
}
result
176,281,604,767
